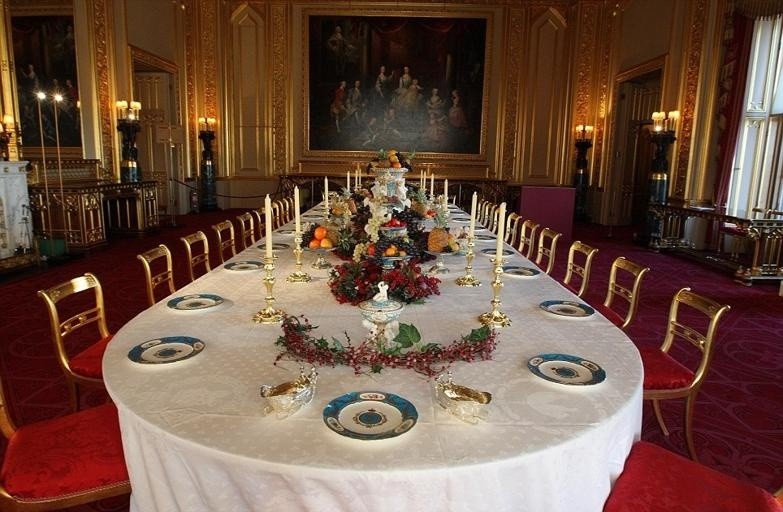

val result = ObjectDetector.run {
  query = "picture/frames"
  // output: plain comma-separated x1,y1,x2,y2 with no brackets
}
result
0,1,82,165
298,6,495,163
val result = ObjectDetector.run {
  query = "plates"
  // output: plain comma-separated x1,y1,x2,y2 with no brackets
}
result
539,301,594,317
224,261,264,272
322,390,418,441
167,294,223,310
447,204,497,242
503,266,541,277
128,336,206,364
276,201,332,234
527,354,606,385
480,249,515,257
256,243,290,251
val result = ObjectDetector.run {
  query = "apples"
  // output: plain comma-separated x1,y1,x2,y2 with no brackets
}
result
368,245,376,256
427,209,437,217
388,150,401,168
309,226,333,250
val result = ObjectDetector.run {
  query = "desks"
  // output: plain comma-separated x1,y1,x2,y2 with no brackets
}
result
278,170,507,208
26,178,161,255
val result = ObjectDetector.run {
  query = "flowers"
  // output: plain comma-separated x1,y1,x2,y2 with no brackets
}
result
329,246,442,306
272,310,514,381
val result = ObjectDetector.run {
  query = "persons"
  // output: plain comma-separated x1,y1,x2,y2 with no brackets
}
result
330,66,467,151
17,64,79,143
326,26,353,76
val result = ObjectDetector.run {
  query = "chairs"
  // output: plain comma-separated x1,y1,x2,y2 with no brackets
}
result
39,273,115,415
631,287,731,462
0,371,132,512
473,200,650,331
136,197,297,308
603,441,782,512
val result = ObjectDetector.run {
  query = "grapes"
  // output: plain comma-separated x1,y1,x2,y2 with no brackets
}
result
396,152,413,171
375,209,427,267
301,223,319,248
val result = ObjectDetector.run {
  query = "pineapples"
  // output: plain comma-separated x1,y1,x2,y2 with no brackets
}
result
327,231,342,246
344,198,357,214
428,228,448,253
416,203,428,217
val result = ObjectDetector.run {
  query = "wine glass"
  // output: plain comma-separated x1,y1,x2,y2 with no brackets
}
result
367,166,415,269
423,247,457,274
359,281,403,340
302,246,339,268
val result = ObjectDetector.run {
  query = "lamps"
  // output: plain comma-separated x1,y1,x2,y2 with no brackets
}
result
0,113,18,161
627,109,680,251
573,123,594,224
197,117,217,213
114,99,142,182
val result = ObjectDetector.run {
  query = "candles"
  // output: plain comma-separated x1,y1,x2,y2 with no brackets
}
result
293,185,300,236
293,168,478,233
470,192,477,236
